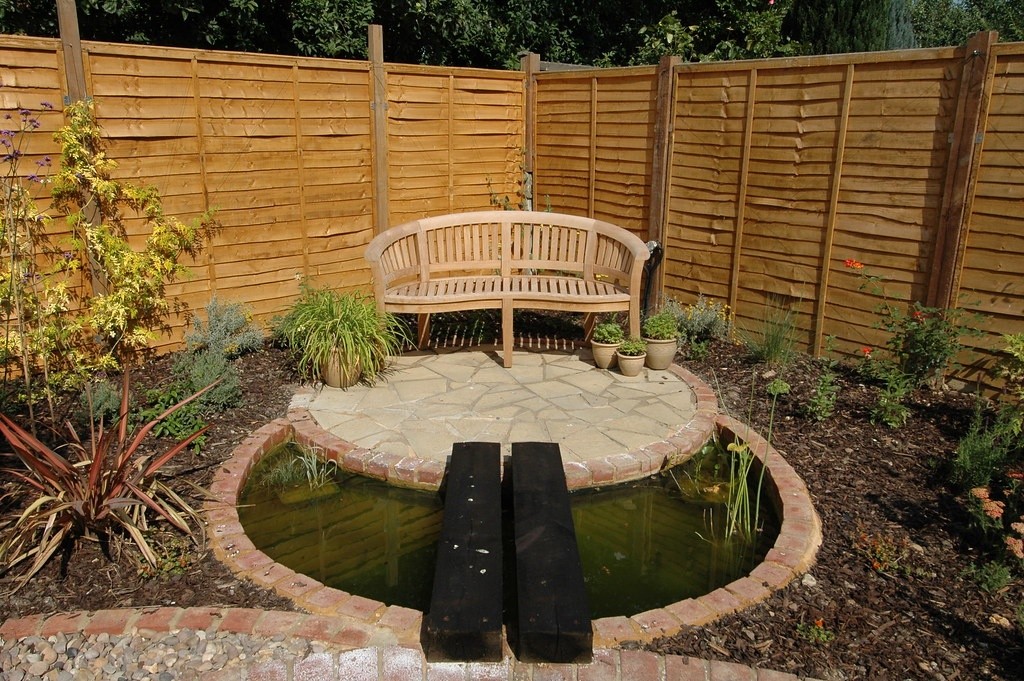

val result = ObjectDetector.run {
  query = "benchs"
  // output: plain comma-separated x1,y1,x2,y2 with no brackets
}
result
365,211,650,368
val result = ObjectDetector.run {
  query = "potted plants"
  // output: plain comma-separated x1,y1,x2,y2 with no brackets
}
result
591,322,626,368
618,337,649,376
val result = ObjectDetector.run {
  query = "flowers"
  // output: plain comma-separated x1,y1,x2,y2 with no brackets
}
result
280,270,400,381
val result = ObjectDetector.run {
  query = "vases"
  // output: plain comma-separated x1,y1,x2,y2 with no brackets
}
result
641,304,679,371
318,343,372,387
